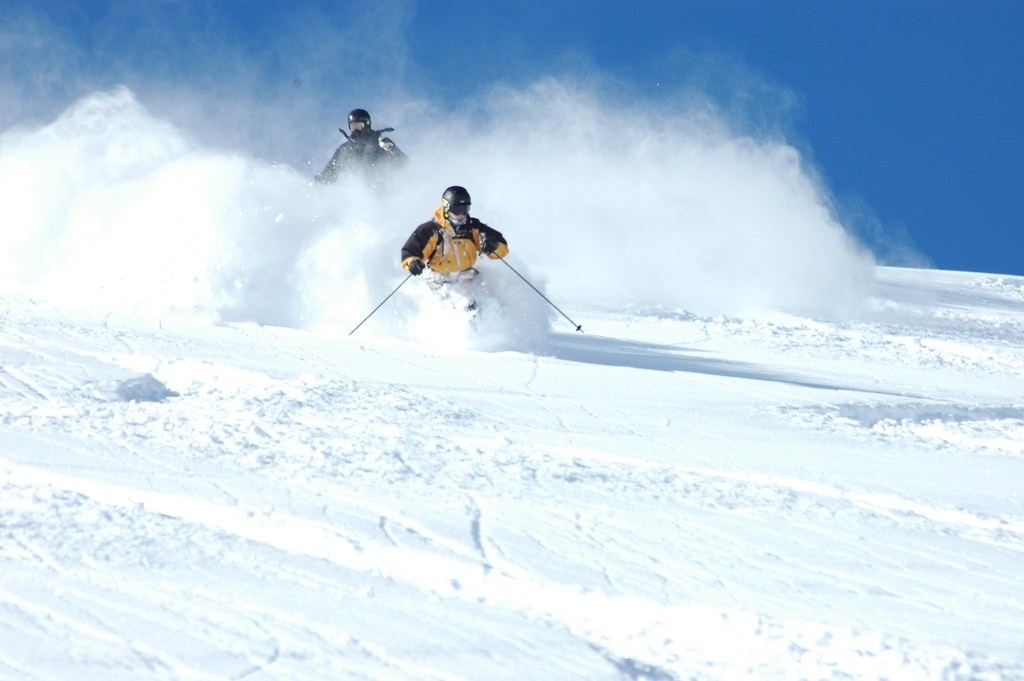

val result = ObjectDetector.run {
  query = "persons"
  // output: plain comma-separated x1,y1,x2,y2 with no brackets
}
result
401,186,509,317
315,108,406,185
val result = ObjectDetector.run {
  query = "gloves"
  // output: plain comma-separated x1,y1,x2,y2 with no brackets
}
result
408,259,425,275
376,138,395,152
478,232,498,256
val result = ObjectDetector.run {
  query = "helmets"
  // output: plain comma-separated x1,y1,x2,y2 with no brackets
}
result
347,109,371,137
442,185,472,228
